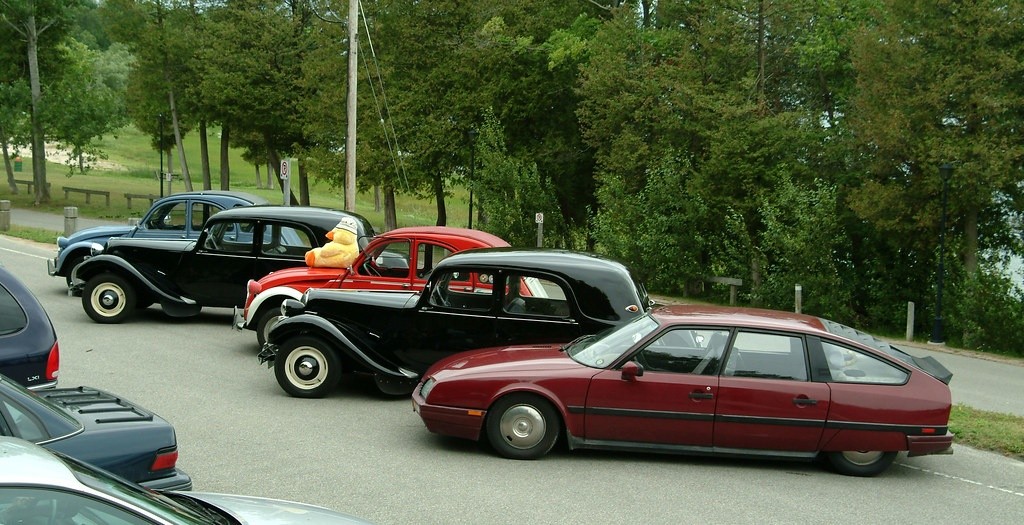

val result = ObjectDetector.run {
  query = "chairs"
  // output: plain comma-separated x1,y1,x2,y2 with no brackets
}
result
690,332,739,377
824,350,847,381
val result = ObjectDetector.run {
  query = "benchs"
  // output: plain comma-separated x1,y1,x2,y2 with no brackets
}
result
505,297,526,314
265,245,287,255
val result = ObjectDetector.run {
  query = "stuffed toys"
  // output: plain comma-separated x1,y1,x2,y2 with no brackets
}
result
305,217,359,268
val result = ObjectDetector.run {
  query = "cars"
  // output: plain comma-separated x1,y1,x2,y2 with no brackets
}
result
67,204,410,322
0,267,60,390
45,190,306,297
0,437,382,525
411,304,954,479
1,374,193,491
257,247,703,399
232,225,550,351
